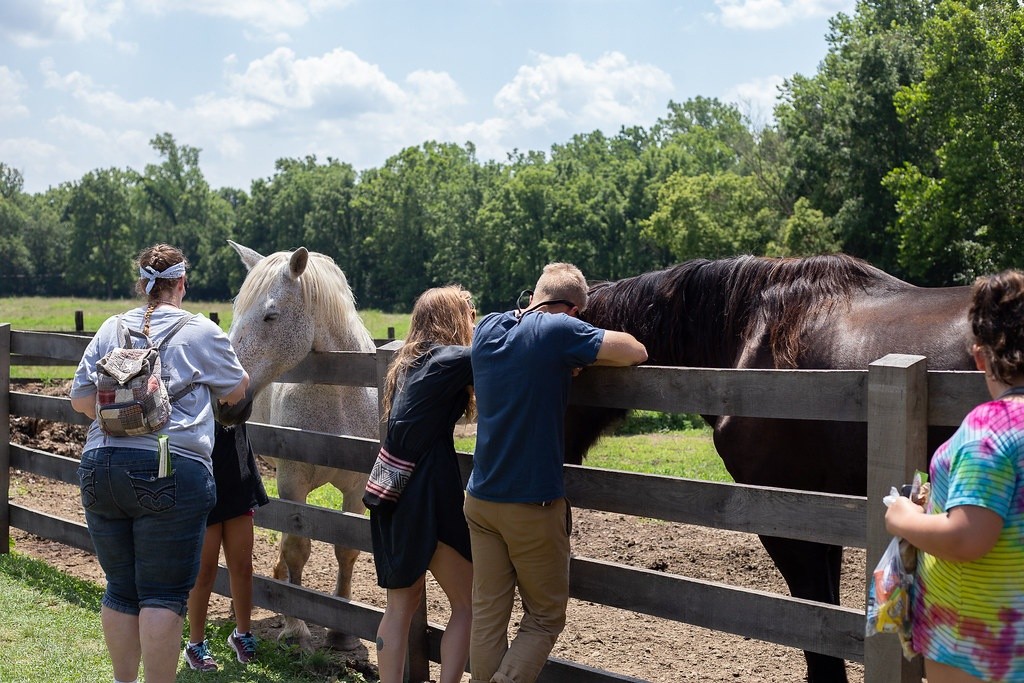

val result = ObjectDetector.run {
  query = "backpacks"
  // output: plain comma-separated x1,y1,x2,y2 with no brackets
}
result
97,312,197,438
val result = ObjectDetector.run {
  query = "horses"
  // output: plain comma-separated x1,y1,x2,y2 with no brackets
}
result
565,253,977,683
212,239,380,655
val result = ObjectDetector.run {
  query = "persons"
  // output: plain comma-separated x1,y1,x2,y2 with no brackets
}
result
461,260,647,682
366,285,475,683
68,244,248,683
181,420,260,673
882,269,1024,683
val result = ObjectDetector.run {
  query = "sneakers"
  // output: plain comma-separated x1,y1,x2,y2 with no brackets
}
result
184,641,218,672
228,628,256,662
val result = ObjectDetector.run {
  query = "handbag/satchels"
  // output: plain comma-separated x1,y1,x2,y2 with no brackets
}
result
865,536,913,637
364,439,424,512
